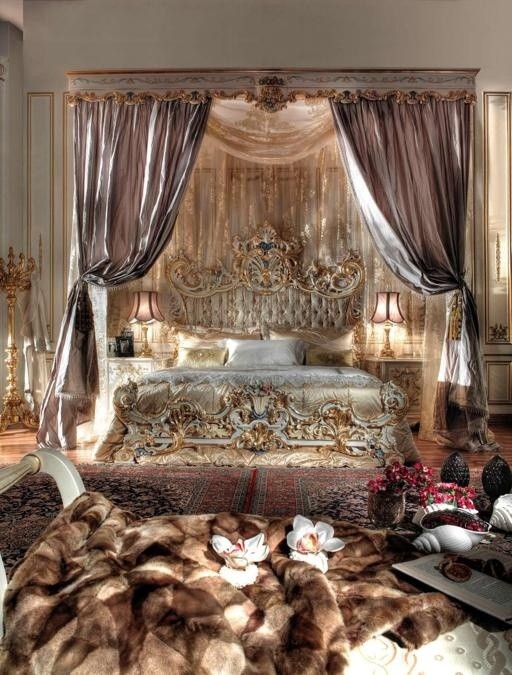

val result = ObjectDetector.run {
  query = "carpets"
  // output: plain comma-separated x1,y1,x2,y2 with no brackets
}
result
0,465,512,582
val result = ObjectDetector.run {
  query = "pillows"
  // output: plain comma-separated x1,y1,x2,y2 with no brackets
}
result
171,324,361,367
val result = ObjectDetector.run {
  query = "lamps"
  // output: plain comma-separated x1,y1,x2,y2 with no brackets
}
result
369,291,406,360
126,290,166,358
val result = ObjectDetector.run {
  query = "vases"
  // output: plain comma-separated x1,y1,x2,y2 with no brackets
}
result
367,489,406,530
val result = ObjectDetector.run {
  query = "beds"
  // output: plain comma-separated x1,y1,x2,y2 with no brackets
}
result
91,327,421,467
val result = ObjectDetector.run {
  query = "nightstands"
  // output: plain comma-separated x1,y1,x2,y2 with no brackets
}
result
105,356,171,423
366,358,425,427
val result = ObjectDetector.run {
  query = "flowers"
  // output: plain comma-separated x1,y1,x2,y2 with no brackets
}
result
365,460,429,511
417,481,480,509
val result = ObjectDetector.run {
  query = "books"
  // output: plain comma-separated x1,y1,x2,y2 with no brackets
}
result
391,553,512,626
